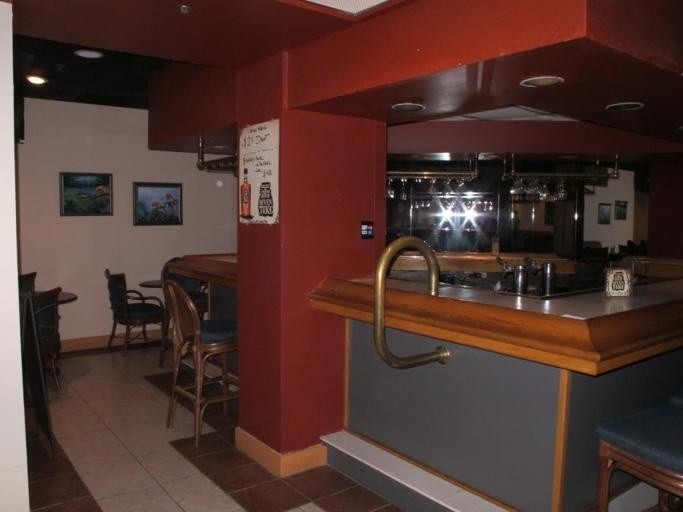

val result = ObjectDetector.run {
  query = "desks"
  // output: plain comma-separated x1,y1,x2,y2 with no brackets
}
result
139,280,163,288
57,292,78,304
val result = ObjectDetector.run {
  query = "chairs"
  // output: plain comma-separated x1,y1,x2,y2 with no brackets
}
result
595,396,683,512
104,268,166,356
20,286,63,393
163,280,240,446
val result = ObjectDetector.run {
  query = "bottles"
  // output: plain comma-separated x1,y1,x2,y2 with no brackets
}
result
542,262,557,295
513,264,530,294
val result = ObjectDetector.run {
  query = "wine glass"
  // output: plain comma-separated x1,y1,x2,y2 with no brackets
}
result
387,176,571,202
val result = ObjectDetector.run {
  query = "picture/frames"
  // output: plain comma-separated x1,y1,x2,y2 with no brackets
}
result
133,181,183,225
59,171,113,216
